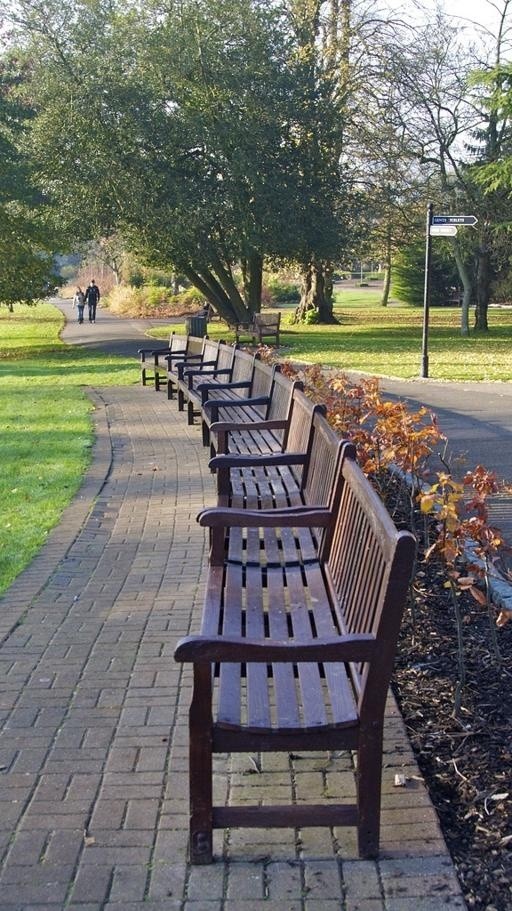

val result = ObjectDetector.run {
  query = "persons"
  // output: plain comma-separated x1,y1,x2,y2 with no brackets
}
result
195,299,211,319
82,279,100,324
72,285,89,324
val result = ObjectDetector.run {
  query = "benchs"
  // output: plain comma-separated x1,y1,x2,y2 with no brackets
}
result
233,312,282,348
173,442,416,862
136,329,352,523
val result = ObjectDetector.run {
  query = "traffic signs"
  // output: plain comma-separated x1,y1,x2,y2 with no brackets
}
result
432,215,478,226
429,225,458,237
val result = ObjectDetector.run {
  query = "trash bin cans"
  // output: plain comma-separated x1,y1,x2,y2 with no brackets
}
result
185,316,207,338
